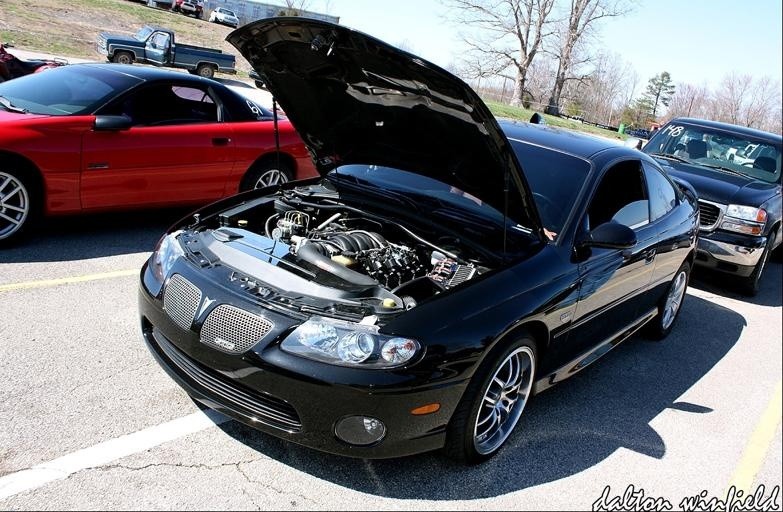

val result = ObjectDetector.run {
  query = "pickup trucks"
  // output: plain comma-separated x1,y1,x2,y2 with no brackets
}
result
97,26,236,78
625,118,783,297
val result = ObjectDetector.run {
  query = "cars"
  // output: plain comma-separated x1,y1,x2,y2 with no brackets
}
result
0,62,317,247
209,8,239,27
136,17,699,466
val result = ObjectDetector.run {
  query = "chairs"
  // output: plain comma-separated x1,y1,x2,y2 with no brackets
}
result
684,140,707,161
752,156,777,174
